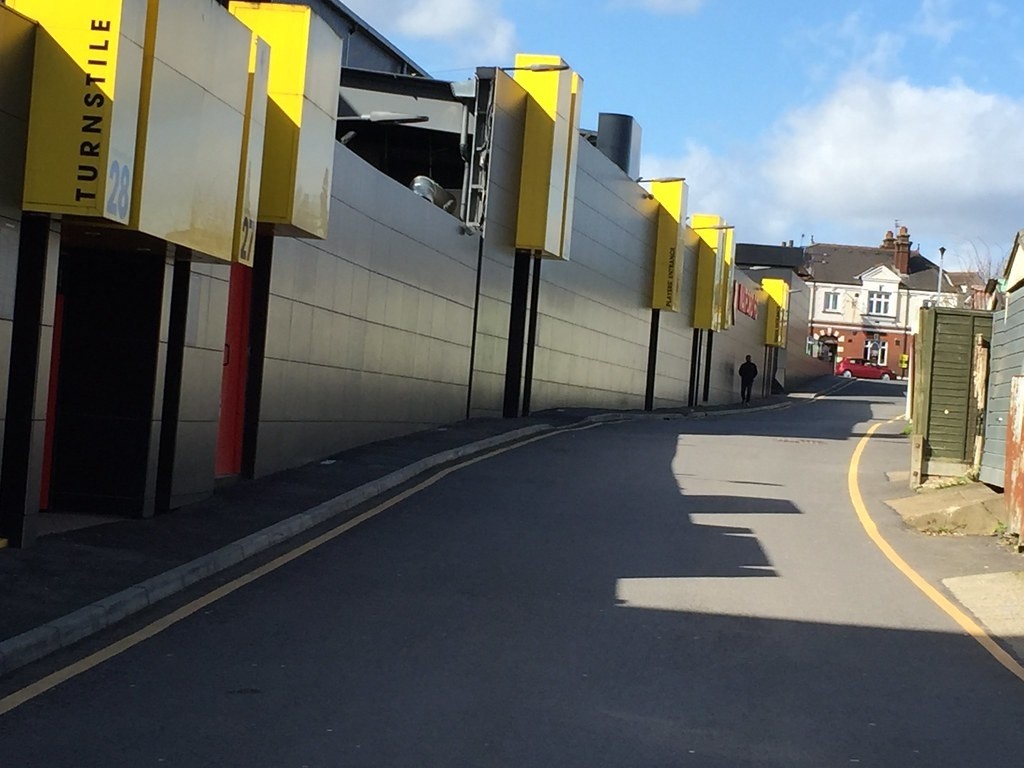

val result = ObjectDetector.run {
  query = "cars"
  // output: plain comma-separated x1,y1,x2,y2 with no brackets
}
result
835,357,896,381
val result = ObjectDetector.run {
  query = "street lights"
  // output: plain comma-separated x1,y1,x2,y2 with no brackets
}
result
936,247,946,308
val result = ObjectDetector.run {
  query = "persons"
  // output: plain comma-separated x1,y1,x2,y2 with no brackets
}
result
738,355,758,407
